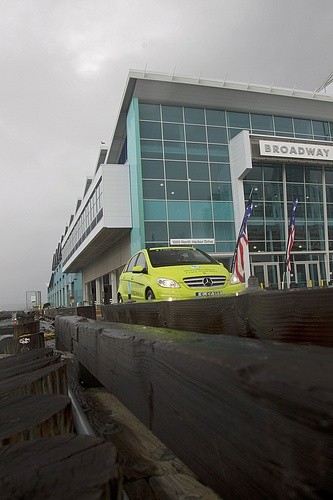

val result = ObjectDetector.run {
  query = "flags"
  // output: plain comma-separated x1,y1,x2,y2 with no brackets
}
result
236,190,254,285
285,192,298,271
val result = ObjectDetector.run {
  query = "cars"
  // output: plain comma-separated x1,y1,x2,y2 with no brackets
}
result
116,246,245,302
